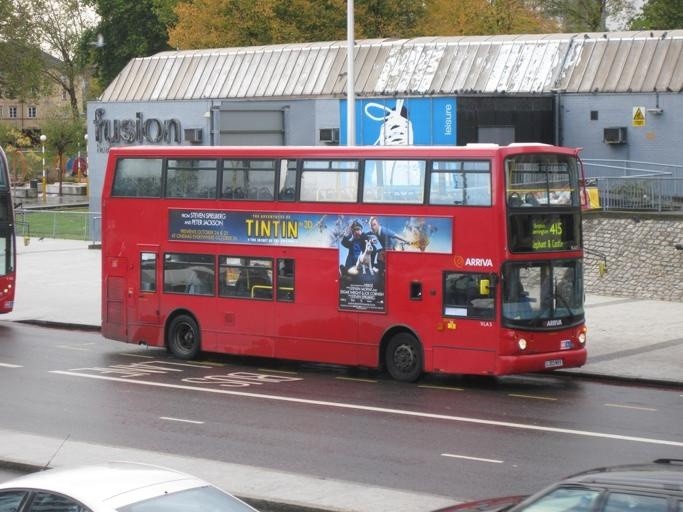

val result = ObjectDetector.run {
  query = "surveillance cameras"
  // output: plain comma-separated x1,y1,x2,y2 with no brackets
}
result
648,109,663,114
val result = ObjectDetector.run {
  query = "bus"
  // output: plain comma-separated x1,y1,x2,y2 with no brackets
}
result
0,145,30,314
100,142,609,383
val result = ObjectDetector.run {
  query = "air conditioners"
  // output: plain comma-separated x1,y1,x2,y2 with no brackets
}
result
320,128,339,143
183,128,202,142
603,126,627,144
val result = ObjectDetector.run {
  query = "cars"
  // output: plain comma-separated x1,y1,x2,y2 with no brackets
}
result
430,457,683,512
1,432,259,512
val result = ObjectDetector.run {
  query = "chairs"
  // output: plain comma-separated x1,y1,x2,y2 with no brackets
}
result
198,186,295,200
319,189,417,200
509,192,536,207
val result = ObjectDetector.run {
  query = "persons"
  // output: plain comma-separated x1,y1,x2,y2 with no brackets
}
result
503,266,530,302
340,217,412,271
549,191,572,206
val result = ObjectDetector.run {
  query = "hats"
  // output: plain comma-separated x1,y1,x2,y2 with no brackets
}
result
351,221,363,229
